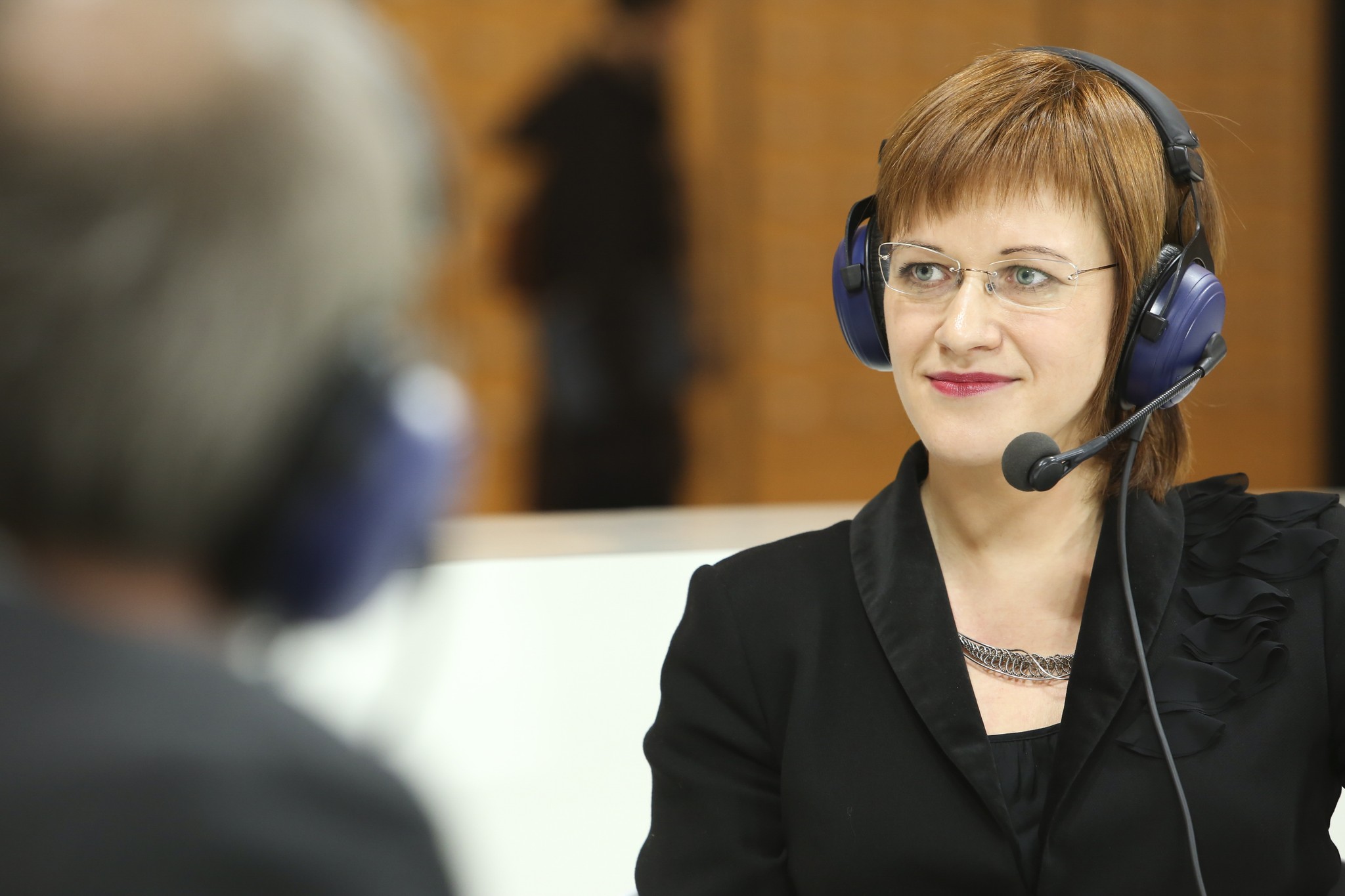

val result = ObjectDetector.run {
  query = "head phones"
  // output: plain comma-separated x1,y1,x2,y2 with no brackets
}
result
830,45,1230,410
216,315,465,619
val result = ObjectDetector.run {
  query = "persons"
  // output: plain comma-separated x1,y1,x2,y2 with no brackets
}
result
3,0,457,896
479,0,701,511
633,44,1345,896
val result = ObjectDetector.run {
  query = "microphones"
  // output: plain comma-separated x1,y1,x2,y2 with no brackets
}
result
1000,336,1229,491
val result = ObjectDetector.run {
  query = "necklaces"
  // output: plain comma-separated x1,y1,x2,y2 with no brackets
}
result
956,630,1075,682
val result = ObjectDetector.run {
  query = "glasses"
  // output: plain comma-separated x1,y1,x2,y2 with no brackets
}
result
876,243,1121,309
